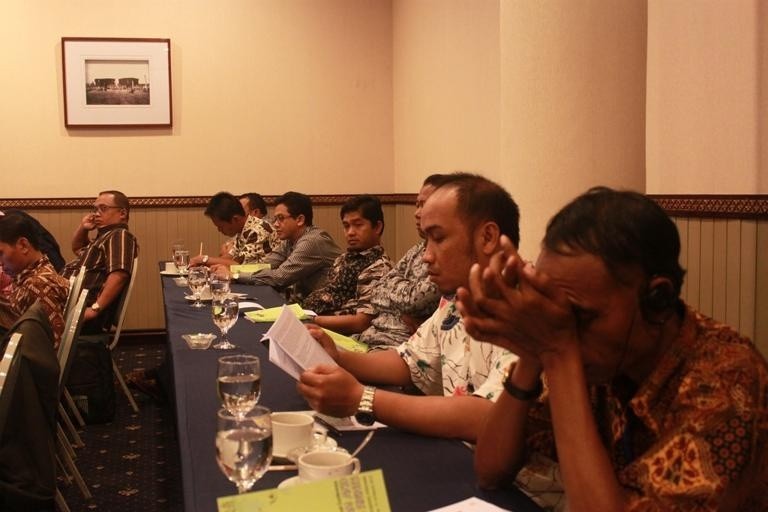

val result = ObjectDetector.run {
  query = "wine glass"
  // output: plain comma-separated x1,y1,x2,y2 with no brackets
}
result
214,405,272,495
215,353,261,423
169,241,240,351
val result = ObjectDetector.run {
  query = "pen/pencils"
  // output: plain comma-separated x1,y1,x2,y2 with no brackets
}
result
267,465,300,470
200,241,203,256
239,297,259,301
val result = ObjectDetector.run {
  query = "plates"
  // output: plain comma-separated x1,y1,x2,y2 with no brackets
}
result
159,270,190,275
276,474,298,490
272,432,337,463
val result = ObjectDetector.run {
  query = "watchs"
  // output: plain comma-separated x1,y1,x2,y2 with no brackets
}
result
91,301,102,313
202,254,208,266
355,385,376,426
233,270,240,283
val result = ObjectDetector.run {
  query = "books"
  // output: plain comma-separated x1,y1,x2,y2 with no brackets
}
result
243,303,307,323
260,304,338,380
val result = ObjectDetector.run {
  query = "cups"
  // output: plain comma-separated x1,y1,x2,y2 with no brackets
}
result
165,262,176,272
298,452,361,480
264,411,326,456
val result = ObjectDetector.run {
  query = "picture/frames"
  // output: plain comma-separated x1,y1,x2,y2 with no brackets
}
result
59,35,173,131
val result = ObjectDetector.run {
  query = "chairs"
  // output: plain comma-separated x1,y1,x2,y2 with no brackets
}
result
0,256,141,511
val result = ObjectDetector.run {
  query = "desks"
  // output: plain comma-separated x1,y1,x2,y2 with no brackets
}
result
158,258,546,512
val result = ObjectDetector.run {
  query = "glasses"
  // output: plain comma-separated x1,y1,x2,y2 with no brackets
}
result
271,212,299,222
88,203,129,213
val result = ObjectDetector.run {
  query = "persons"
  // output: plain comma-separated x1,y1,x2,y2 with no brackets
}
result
346,174,446,352
209,191,343,303
220,193,276,255
454,185,767,511
295,173,521,445
300,194,394,334
60,189,135,334
1,214,71,351
187,190,282,270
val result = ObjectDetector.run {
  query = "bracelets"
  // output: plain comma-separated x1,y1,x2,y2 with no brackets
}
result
311,315,316,321
501,362,542,401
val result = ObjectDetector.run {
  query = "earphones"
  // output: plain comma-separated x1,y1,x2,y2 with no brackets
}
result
648,285,670,315
